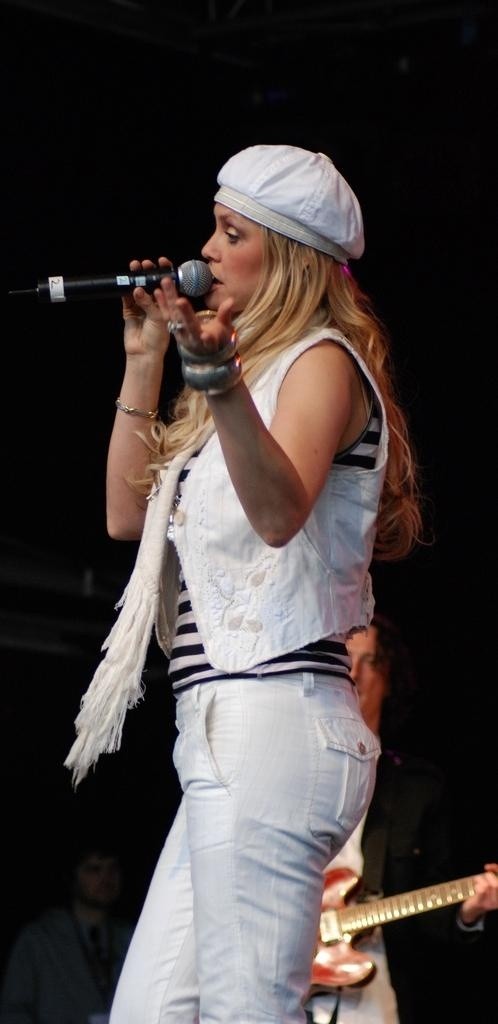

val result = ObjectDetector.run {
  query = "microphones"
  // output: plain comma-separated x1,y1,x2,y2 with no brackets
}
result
37,259,212,303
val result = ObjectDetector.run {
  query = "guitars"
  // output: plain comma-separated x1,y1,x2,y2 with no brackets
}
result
302,867,498,1003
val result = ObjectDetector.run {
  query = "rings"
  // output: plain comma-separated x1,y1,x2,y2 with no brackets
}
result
166,319,186,330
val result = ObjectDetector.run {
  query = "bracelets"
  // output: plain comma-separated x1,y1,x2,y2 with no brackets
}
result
114,395,162,423
178,352,245,396
171,307,248,364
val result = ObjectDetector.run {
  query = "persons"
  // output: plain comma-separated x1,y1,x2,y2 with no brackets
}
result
309,613,498,1023
1,833,146,1024
58,137,438,1023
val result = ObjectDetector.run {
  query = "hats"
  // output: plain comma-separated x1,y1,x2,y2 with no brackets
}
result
209,142,367,264
